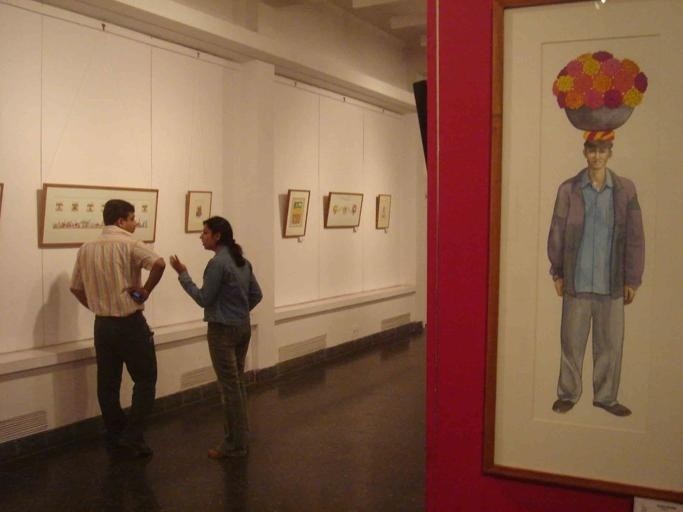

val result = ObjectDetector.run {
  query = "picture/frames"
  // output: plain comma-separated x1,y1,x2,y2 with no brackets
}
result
185,189,215,234
480,0,683,502
376,194,391,229
281,188,311,238
323,191,365,229
38,182,160,248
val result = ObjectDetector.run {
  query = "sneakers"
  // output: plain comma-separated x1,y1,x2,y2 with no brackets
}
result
105,429,153,456
207,437,248,457
593,400,632,416
552,400,575,414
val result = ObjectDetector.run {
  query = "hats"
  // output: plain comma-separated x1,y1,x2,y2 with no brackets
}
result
585,140,614,148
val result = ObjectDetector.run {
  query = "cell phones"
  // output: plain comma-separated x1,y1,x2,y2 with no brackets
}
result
130,290,144,302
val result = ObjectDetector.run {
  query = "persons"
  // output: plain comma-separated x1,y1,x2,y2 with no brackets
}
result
170,216,262,459
549,130,646,416
70,199,166,457
